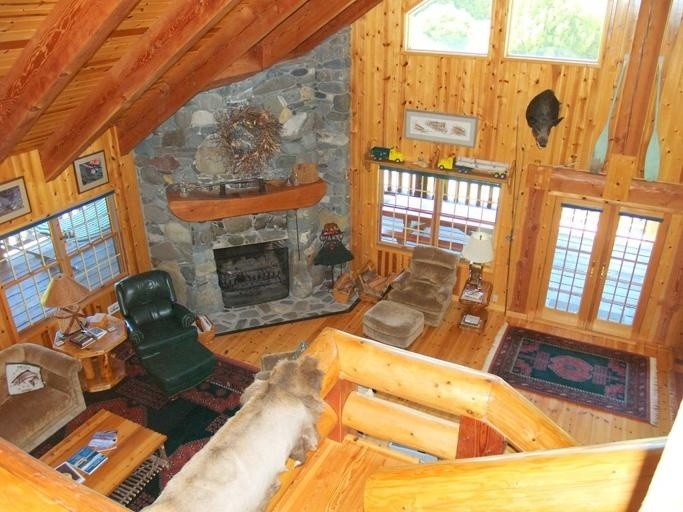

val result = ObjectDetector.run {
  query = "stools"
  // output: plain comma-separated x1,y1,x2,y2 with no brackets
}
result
360,300,423,349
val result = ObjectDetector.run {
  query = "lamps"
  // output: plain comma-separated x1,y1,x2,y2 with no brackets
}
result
461,231,493,286
40,274,89,335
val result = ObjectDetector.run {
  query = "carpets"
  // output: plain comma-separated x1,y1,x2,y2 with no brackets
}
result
665,367,683,422
481,322,659,428
26,341,261,511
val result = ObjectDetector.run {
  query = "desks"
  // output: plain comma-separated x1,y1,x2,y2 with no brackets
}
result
51,313,128,393
459,278,492,336
38,407,171,507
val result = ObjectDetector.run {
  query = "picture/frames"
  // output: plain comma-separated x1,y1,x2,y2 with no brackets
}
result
403,108,477,148
71,149,109,195
0,175,32,226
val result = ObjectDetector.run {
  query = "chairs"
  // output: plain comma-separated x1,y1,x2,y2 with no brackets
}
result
387,245,459,328
114,269,216,398
0,342,86,454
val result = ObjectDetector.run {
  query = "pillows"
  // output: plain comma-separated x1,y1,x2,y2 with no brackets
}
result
5,361,45,396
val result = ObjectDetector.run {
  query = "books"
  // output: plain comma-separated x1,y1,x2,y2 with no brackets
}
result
66,446,108,475
68,326,108,351
87,429,117,452
55,461,85,483
